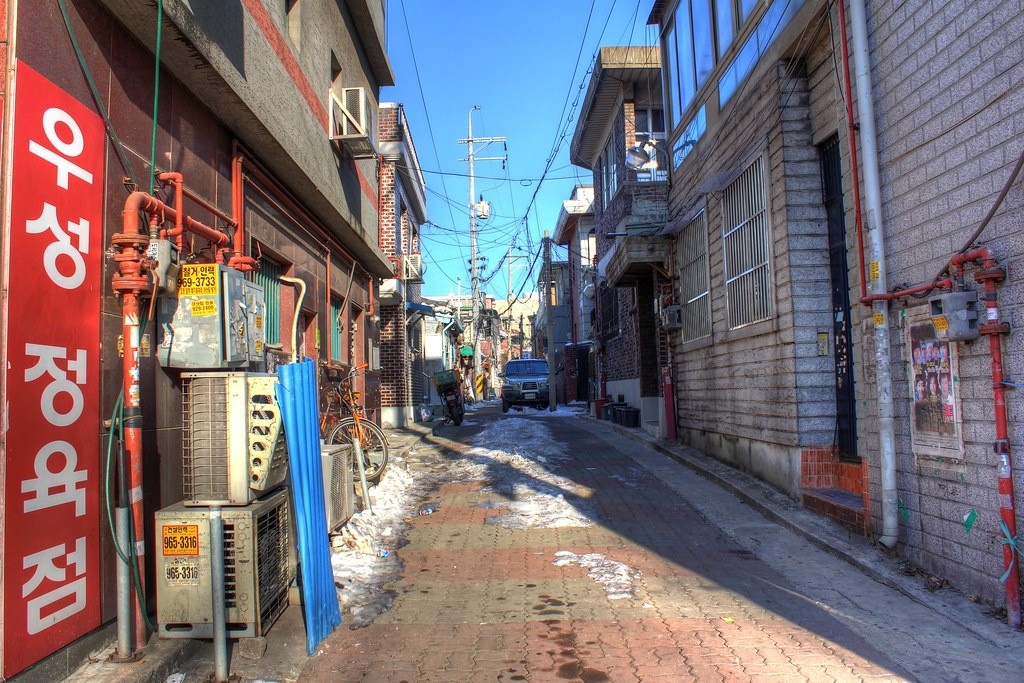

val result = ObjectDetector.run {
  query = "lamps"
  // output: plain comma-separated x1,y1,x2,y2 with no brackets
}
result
625,137,671,185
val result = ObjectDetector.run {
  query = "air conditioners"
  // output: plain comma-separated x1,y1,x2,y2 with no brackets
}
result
329,87,380,160
154,487,290,639
323,444,355,534
404,253,426,284
181,371,288,508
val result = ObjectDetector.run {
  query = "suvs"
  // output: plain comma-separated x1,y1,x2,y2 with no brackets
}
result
498,358,550,412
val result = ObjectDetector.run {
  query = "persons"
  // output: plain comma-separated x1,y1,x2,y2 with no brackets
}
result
911,343,952,403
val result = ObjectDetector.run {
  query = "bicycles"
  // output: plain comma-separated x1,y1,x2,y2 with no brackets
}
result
326,364,390,482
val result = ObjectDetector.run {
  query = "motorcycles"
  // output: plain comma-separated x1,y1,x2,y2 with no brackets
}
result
429,368,466,427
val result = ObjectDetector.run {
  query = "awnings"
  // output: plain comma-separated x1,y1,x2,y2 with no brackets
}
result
460,346,473,357
407,302,436,319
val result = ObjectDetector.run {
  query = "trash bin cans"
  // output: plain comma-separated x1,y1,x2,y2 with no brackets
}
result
595,393,640,427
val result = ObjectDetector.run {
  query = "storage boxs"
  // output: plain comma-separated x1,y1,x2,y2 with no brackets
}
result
600,402,639,427
